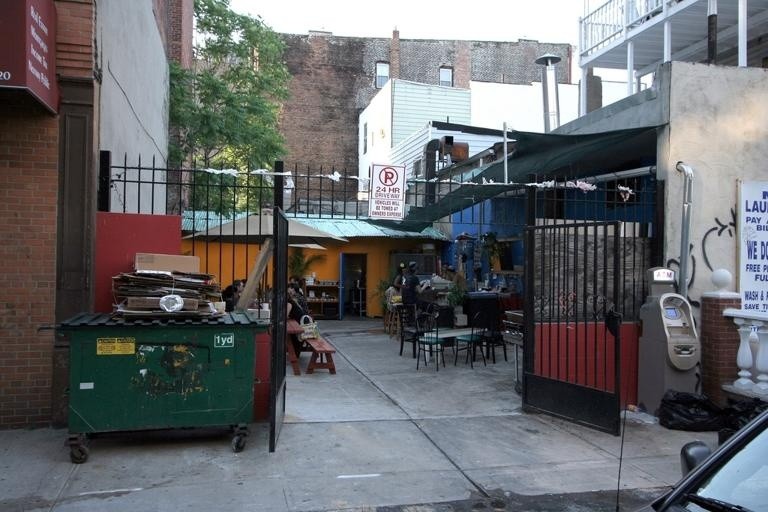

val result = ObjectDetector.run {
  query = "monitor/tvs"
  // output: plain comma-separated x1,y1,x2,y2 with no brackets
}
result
666,308,677,318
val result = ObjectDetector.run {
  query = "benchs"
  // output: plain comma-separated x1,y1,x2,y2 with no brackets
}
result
304,334,337,376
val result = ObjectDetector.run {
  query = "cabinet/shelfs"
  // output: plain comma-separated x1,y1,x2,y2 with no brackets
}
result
300,276,341,319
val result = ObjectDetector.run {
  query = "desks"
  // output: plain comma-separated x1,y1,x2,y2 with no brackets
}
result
284,318,305,377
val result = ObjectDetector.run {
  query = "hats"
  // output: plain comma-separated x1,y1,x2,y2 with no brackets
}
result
409,262,417,270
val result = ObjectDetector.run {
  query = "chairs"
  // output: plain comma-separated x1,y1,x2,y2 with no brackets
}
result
397,301,507,375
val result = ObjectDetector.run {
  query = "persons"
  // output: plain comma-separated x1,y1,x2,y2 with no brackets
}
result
438,262,461,283
287,276,309,358
393,261,429,304
222,279,247,312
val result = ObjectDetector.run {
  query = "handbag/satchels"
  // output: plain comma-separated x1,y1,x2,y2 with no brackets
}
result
296,315,319,342
